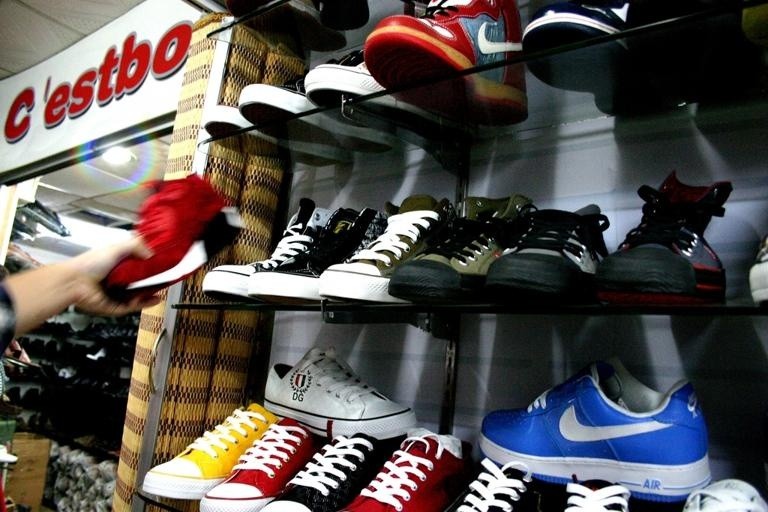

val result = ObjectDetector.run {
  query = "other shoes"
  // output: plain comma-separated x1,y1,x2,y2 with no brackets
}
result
748,233,768,306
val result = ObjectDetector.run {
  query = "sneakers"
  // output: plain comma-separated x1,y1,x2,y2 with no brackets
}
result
683,479,768,512
264,346,418,441
487,205,611,304
248,208,391,304
107,174,246,289
598,172,734,306
522,0,628,115
237,74,404,157
205,104,352,169
339,427,473,512
259,433,381,512
387,196,534,303
205,198,336,306
564,475,631,512
303,48,446,141
320,195,451,306
10,200,70,241
200,418,311,512
142,403,280,500
0,257,141,512
362,0,526,131
479,361,713,502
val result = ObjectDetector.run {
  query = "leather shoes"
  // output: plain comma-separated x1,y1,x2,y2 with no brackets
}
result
444,457,541,512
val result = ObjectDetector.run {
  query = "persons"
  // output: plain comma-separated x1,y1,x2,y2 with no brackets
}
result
0,262,22,396
0,227,164,356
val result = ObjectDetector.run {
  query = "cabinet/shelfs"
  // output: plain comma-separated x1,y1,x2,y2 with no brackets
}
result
0,1,768,512
